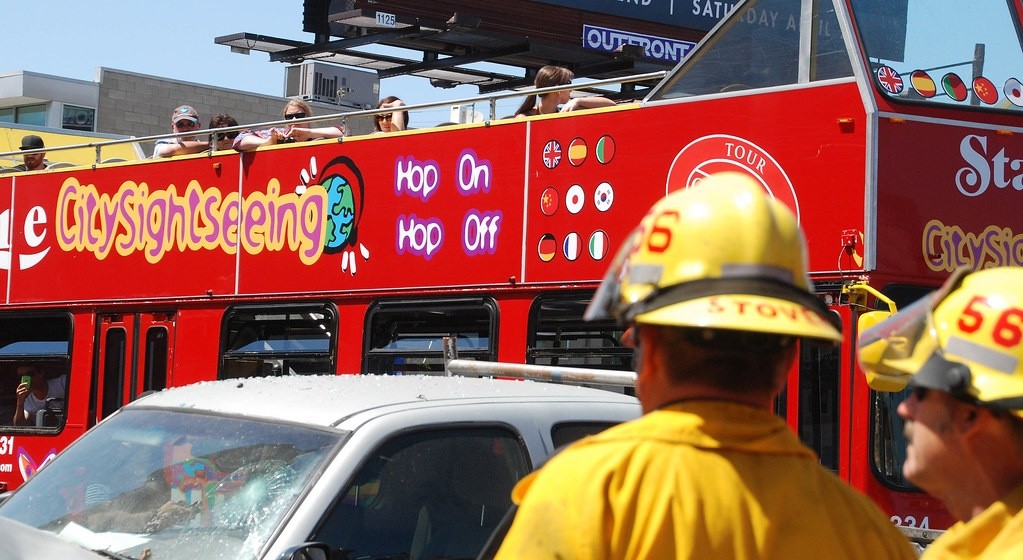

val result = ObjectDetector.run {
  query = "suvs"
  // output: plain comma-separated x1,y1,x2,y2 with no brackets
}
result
1,359,641,559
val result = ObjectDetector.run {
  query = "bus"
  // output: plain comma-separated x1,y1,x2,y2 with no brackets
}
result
0,0,1023,560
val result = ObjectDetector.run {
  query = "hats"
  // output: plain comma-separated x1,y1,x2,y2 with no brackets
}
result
18,135,43,150
171,105,199,123
858,266,1023,425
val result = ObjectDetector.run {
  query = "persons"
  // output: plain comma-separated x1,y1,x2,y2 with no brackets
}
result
515,65,617,119
200,112,241,153
11,134,50,173
153,104,209,158
368,96,409,135
897,264,1023,560
231,98,351,152
493,171,920,560
12,359,67,428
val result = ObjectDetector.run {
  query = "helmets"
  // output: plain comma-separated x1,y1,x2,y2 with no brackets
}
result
617,173,841,341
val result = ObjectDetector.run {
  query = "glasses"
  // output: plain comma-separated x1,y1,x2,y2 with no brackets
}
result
284,113,305,120
175,121,194,128
375,113,392,122
16,370,34,381
216,131,239,141
915,385,928,401
